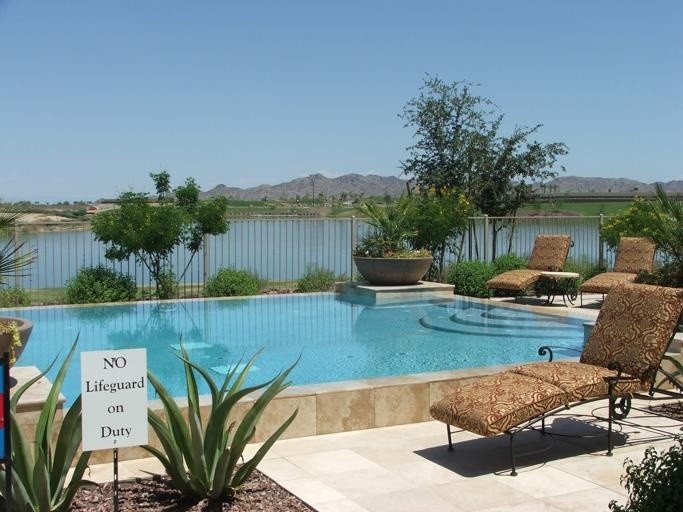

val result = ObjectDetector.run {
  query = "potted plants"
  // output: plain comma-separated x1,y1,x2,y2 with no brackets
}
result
0,315,33,369
348,193,433,284
352,305,431,373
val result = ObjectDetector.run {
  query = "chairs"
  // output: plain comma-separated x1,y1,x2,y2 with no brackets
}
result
579,238,658,307
431,283,682,478
486,233,571,302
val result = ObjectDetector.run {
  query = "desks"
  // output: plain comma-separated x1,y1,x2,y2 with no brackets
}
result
540,272,580,308
